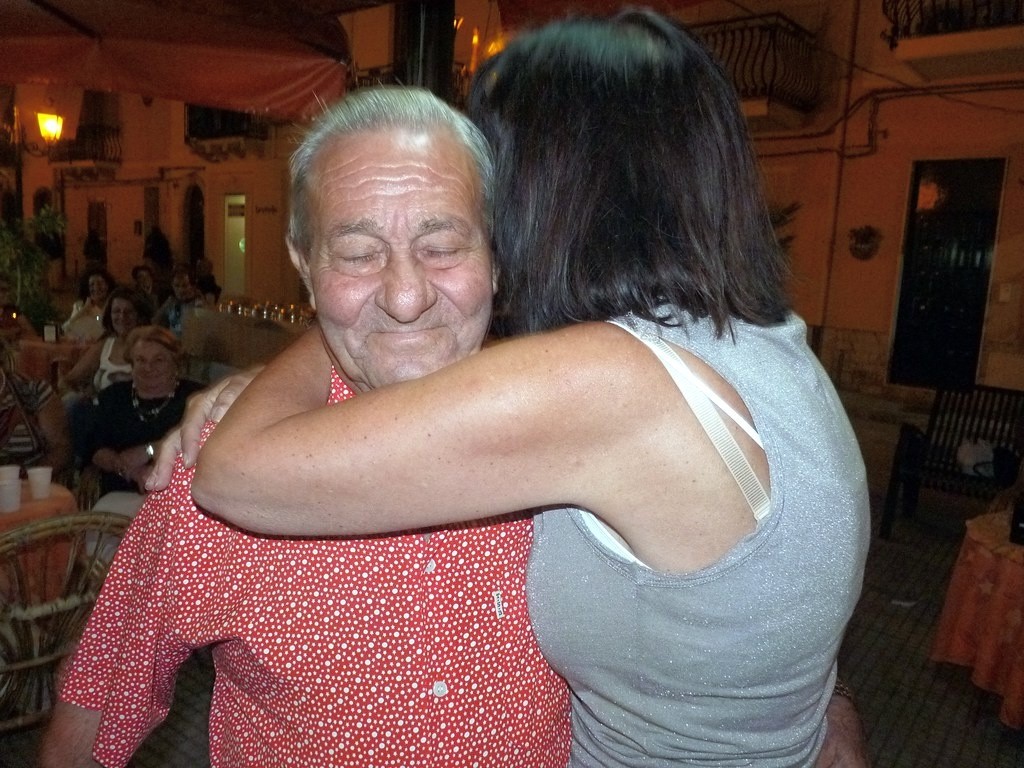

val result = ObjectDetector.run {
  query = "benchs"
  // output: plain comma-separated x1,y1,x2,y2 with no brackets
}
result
879,382,1024,540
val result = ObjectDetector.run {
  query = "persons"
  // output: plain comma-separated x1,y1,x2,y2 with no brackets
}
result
193,257,216,297
35,85,871,768
0,338,66,479
151,271,204,335
69,266,115,340
143,6,871,768
133,264,158,308
68,287,151,394
73,325,194,498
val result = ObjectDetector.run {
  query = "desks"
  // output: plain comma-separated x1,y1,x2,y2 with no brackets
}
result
181,302,309,385
930,509,1024,729
1,479,79,713
18,337,99,392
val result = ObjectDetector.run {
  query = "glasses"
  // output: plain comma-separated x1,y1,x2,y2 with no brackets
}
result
106,309,137,317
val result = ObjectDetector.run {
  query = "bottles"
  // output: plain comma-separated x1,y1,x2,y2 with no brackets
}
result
219,300,304,325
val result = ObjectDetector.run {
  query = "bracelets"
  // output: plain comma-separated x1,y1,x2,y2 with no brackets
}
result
832,679,851,700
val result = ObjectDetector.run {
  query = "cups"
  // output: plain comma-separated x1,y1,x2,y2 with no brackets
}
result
0,464,22,514
27,467,53,500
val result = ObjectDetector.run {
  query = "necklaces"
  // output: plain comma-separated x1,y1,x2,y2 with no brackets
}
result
131,375,180,422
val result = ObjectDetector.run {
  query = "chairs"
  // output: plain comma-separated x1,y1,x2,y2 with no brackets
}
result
1,511,133,731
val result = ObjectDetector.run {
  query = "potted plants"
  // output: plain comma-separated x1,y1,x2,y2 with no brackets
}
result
848,225,878,260
